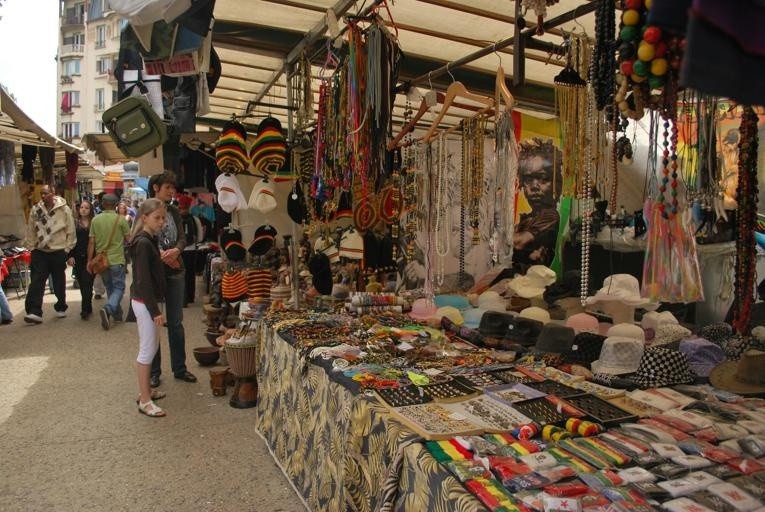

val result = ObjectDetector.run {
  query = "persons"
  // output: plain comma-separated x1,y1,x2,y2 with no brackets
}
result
0,232,15,324
22,183,79,324
91,192,138,330
175,197,201,307
67,202,99,317
133,174,198,386
128,197,176,418
512,139,567,271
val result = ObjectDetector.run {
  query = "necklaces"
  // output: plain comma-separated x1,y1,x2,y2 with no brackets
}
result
391,56,518,293
281,22,392,223
549,0,765,336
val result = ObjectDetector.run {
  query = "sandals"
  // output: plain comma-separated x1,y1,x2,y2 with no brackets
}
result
139,399,166,419
136,390,166,405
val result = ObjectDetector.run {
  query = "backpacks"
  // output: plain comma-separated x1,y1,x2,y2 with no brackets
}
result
178,212,199,248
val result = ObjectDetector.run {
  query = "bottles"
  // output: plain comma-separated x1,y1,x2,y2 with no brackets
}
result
618,203,626,229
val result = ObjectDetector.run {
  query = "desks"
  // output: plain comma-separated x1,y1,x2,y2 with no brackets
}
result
260,316,765,512
0,253,30,297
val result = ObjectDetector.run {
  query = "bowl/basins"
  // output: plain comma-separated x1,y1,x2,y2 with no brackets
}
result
193,347,219,365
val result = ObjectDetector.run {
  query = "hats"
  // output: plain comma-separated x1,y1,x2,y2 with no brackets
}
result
214,172,279,214
218,223,279,259
212,119,288,175
309,226,366,294
409,264,765,398
286,184,309,226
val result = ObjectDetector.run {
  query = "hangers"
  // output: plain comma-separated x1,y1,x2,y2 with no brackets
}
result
385,68,499,150
187,133,203,147
422,61,496,144
496,36,514,124
317,0,398,85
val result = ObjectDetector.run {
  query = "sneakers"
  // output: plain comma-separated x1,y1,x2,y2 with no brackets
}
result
147,371,161,387
173,367,197,382
113,305,124,324
99,307,111,331
54,306,68,318
22,312,44,324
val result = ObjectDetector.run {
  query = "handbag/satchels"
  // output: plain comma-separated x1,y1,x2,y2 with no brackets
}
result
99,93,169,159
87,251,110,274
